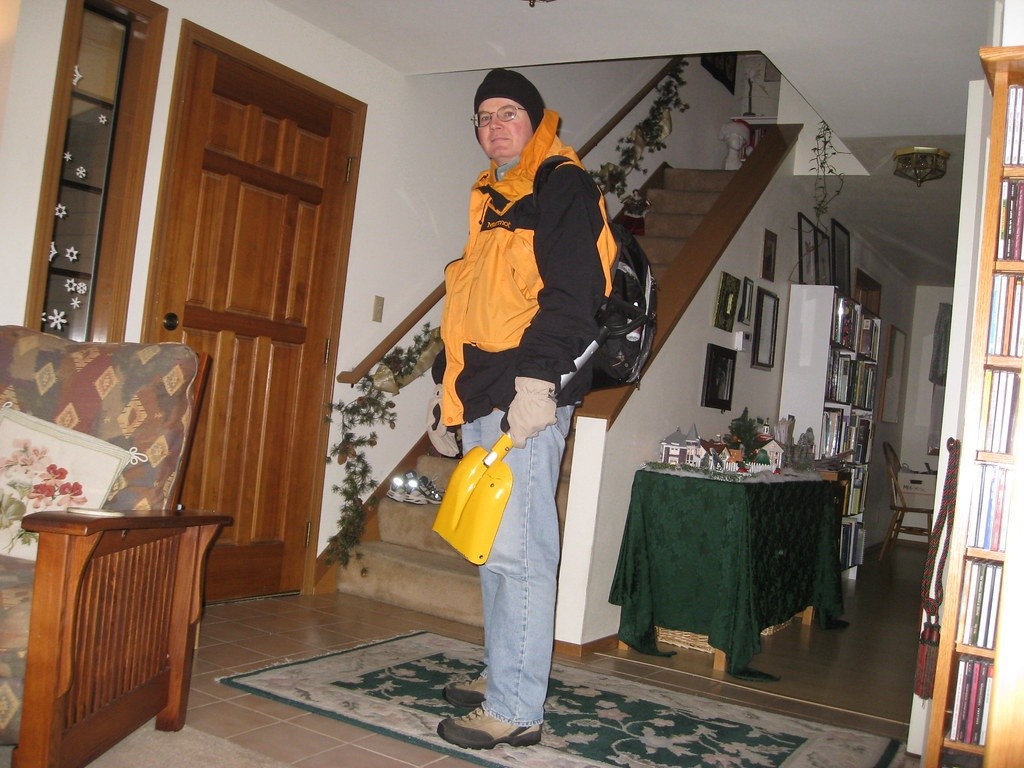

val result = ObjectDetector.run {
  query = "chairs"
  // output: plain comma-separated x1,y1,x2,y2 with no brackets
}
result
879,440,934,560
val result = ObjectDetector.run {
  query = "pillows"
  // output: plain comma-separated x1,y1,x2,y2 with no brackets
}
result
0,406,133,562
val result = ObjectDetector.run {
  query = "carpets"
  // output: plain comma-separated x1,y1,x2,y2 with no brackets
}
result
214,629,911,768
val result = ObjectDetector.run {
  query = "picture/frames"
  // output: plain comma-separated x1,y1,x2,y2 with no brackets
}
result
713,271,741,333
737,277,754,326
701,344,737,411
750,286,780,372
797,211,817,285
760,228,778,283
815,226,832,286
878,324,907,424
832,218,852,298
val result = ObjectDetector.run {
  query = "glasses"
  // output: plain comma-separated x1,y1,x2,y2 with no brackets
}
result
469,105,528,128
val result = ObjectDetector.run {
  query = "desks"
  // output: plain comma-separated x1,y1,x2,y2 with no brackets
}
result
608,463,846,682
730,116,779,162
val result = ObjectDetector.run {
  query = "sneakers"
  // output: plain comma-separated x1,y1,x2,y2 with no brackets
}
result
438,706,544,751
386,470,444,506
445,677,489,708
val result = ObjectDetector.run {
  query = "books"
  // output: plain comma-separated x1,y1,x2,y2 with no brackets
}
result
921,75,1024,758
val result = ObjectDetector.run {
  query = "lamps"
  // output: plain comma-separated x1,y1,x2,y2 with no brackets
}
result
891,145,952,187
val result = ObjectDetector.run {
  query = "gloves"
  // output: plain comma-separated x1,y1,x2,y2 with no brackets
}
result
426,384,463,459
502,378,561,449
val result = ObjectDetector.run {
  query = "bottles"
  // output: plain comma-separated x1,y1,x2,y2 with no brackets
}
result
626,301,641,342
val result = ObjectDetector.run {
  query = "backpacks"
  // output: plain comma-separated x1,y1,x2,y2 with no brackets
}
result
533,155,658,390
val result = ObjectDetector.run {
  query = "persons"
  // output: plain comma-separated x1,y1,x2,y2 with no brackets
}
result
435,64,623,748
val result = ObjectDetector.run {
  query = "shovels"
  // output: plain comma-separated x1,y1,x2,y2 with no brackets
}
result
432,322,613,565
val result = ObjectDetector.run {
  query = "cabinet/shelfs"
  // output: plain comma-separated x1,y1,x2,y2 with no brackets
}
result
923,46,1024,768
778,283,882,580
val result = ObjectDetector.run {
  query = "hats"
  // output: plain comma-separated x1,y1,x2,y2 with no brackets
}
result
473,68,545,144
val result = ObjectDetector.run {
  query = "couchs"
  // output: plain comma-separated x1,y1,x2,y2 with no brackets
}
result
0,326,236,768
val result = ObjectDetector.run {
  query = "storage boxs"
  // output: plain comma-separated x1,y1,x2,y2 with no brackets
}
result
897,471,937,509
